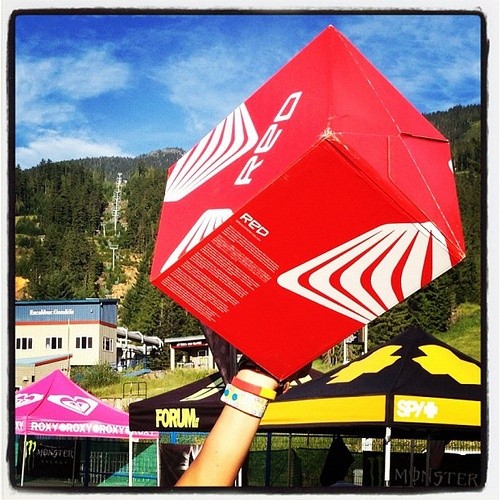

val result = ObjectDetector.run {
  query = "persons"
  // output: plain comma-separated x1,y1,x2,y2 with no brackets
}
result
171,357,314,488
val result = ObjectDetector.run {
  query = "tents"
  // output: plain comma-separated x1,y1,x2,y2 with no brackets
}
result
125,319,484,490
15,369,161,487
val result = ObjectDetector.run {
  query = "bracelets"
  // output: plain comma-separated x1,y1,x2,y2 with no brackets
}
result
220,357,280,420
